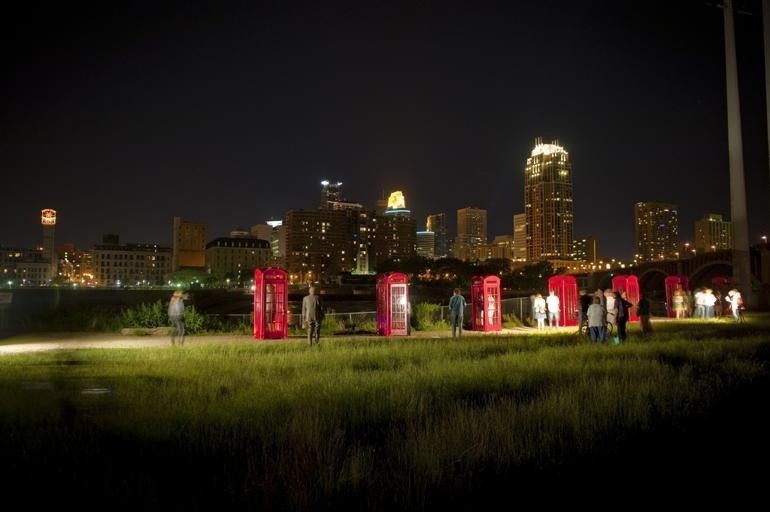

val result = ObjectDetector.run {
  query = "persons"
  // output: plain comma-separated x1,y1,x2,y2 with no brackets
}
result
578,289,593,335
300,286,327,346
449,288,467,337
613,291,633,340
586,295,606,344
546,289,561,329
168,289,191,347
532,293,547,330
672,286,746,322
636,291,650,338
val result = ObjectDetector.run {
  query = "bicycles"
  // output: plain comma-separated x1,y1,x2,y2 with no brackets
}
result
579,311,615,336
